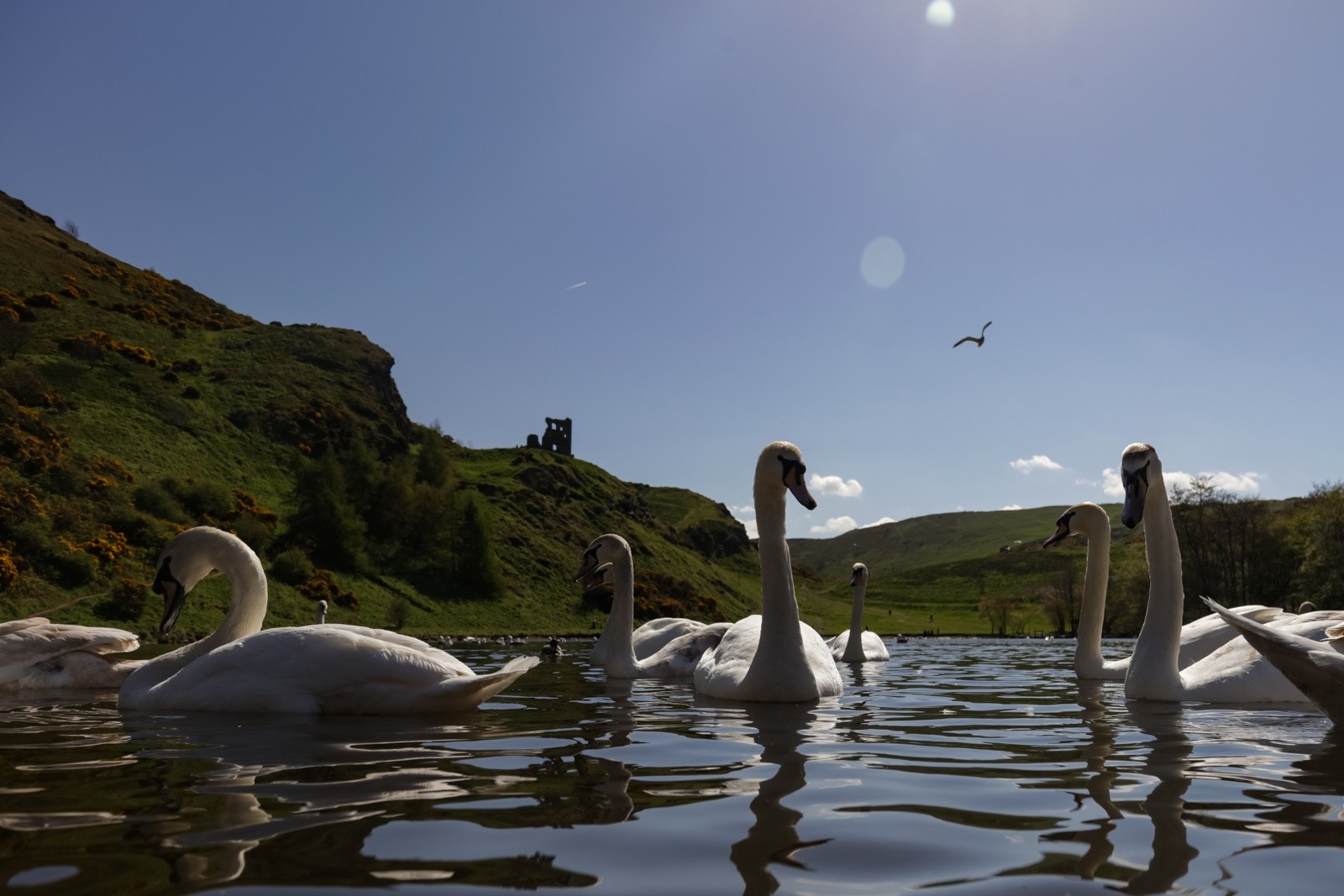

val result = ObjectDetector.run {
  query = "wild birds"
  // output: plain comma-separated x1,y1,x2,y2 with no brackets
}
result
954,321,992,348
312,595,1081,668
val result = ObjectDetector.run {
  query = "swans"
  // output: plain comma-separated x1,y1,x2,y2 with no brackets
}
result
1,616,160,694
1199,592,1344,733
571,531,734,681
833,559,890,663
1120,442,1342,701
691,442,845,705
586,561,712,668
1041,501,1287,683
115,524,540,717
